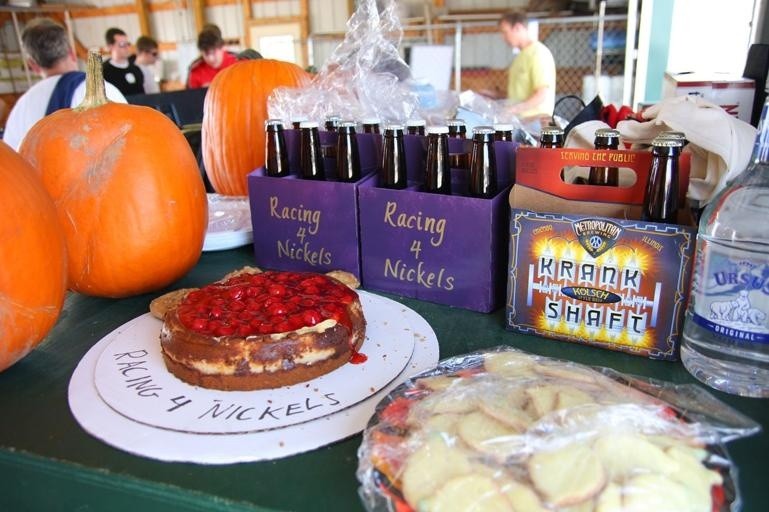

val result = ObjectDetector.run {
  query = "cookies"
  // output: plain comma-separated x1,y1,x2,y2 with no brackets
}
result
210,266,262,285
325,269,361,291
401,350,723,510
149,286,197,321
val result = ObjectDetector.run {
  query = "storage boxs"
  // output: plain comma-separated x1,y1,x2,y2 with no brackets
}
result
663,70,756,130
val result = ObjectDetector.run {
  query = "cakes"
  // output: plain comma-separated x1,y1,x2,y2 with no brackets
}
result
161,269,367,390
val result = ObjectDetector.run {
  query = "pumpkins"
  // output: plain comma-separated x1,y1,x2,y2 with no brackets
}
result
0,138,69,371
199,60,311,195
18,46,210,298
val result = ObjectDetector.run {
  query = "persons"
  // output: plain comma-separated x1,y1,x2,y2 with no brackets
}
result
2,16,130,154
101,27,145,97
477,12,557,129
133,37,164,95
185,22,264,90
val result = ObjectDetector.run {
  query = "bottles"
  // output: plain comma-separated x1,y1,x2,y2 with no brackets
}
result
539,126,684,222
681,74,769,399
263,116,514,198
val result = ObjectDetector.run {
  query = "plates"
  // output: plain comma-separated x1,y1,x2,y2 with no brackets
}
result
204,230,252,252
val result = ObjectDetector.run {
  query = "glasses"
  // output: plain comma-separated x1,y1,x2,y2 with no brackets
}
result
116,42,132,47
145,48,156,56
